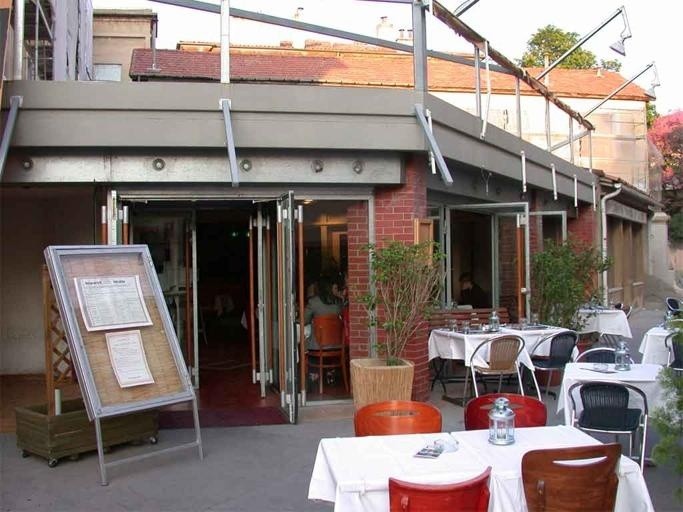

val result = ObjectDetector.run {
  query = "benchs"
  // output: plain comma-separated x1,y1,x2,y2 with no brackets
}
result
427,309,513,393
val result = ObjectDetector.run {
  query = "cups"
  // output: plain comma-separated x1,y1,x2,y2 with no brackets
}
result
518,313,538,328
446,319,470,334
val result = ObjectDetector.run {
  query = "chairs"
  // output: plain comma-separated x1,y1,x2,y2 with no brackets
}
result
569,381,657,466
356,400,442,433
389,466,491,509
523,442,623,512
578,348,633,365
463,335,525,407
616,302,634,319
665,332,681,371
303,313,347,393
665,298,681,321
464,394,548,428
517,331,580,396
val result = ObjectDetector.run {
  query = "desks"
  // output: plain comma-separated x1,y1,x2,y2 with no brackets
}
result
319,425,639,511
640,327,680,366
575,309,633,348
556,363,678,447
427,322,579,406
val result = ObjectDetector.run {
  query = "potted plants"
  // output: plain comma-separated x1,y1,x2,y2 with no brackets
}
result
348,239,456,415
526,234,616,387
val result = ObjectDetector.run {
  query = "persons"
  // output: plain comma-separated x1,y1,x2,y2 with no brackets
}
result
294,270,350,394
458,271,492,309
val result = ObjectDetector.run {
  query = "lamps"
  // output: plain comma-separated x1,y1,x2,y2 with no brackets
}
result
536,5,660,118
